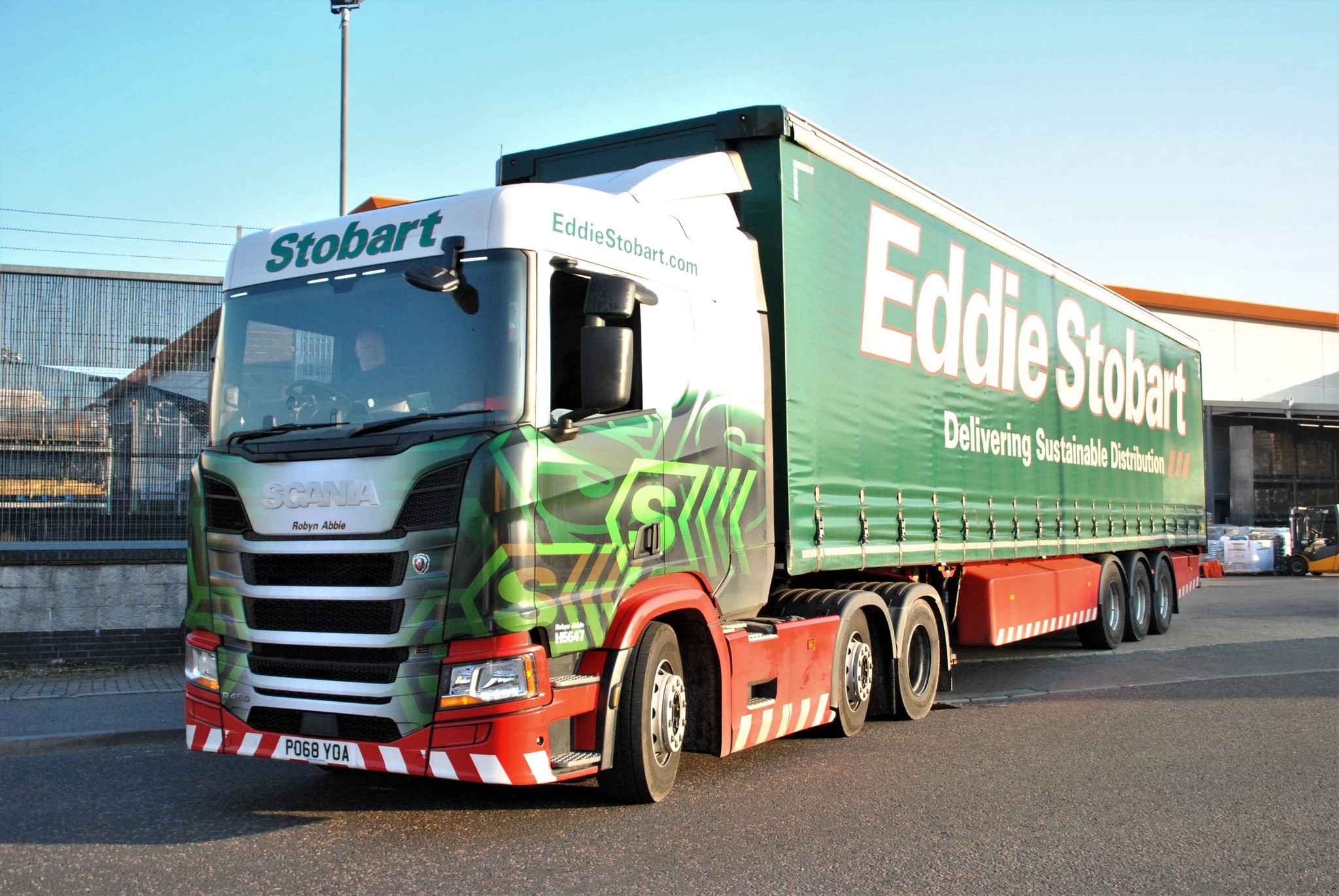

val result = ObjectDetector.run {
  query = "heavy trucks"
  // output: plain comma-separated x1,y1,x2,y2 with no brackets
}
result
184,105,1207,808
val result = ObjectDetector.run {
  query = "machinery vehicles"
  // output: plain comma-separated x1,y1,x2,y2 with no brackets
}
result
1287,504,1339,576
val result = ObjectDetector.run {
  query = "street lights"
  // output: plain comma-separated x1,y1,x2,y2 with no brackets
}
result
331,0,360,218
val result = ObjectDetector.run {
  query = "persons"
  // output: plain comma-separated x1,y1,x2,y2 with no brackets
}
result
285,329,386,401
